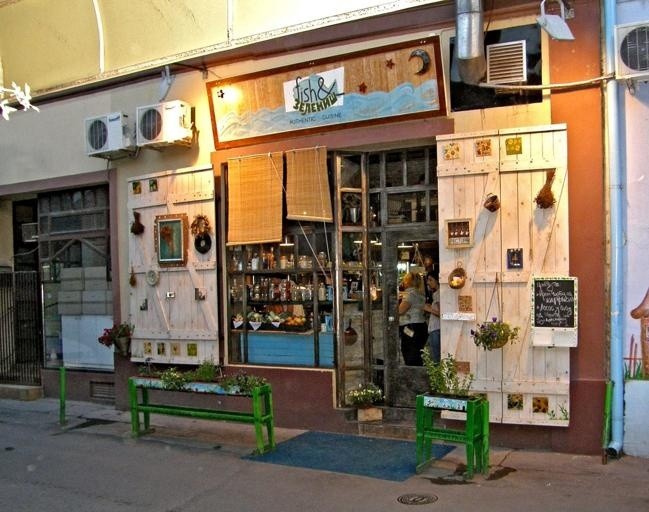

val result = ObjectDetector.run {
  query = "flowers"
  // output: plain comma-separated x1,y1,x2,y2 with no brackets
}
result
471,317,521,351
98,322,135,348
348,385,384,408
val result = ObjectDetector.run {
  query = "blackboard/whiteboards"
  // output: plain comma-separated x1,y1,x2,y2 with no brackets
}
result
531,275,579,348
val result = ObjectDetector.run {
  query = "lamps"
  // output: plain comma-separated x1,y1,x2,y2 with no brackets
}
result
536,0,575,40
158,63,208,103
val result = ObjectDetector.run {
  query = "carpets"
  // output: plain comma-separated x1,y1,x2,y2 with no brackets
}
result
242,430,456,483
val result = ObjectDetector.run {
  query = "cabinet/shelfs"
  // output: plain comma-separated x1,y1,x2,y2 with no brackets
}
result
230,267,361,305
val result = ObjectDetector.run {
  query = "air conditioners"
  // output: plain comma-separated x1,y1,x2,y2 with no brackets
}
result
614,20,649,94
84,111,135,160
136,100,190,148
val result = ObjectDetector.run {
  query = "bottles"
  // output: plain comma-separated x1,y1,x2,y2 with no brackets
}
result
130,211,145,235
230,252,365,304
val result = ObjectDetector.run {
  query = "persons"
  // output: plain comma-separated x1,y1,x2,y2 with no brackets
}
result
422,271,440,367
394,272,429,367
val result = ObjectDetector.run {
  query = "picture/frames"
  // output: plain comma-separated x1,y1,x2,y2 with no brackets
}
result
158,218,183,262
445,218,474,248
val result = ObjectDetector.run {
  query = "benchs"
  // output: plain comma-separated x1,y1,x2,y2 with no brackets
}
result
127,375,275,455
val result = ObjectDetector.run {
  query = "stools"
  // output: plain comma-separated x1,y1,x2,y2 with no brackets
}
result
417,392,489,481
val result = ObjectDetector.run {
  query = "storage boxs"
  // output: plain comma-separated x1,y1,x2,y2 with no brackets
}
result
16,206,113,368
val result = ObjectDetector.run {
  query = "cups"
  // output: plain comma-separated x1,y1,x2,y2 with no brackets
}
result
483,194,501,213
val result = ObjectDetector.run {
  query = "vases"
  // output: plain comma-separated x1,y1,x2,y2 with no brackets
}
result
116,337,129,356
358,409,382,421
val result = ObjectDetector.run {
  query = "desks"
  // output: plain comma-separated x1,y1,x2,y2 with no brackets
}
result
61,315,114,373
